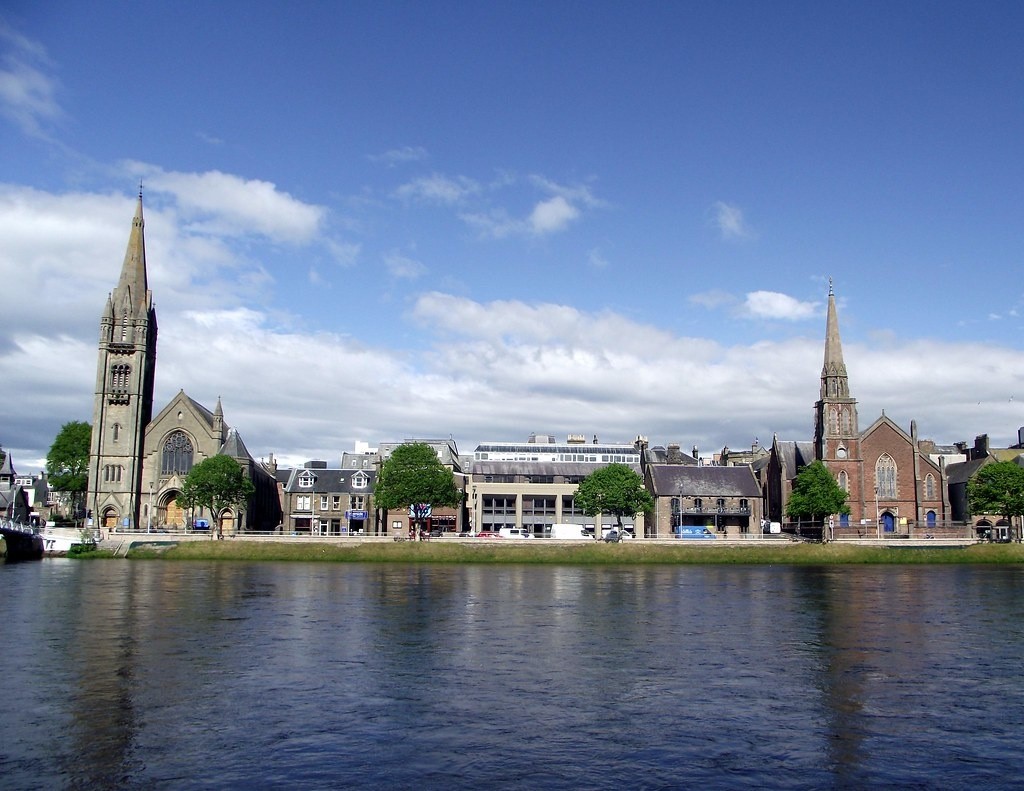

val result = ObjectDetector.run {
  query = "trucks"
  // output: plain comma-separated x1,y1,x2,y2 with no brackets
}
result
763,522,781,533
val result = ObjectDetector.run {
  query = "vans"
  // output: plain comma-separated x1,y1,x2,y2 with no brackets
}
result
551,524,593,539
499,528,536,538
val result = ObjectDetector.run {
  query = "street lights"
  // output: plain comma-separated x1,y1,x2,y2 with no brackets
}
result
472,485,477,537
874,486,880,539
679,486,682,539
11,482,17,522
147,481,155,534
311,484,315,534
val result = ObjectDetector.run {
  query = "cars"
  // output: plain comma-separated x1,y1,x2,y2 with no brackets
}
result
605,530,633,544
478,532,502,538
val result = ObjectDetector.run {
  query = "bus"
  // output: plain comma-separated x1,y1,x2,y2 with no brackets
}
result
676,526,717,540
